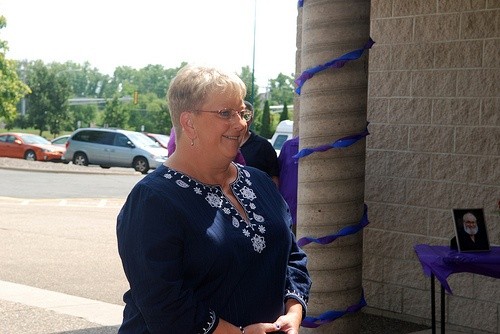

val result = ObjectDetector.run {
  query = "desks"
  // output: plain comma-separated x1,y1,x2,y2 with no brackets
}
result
414,245,500,334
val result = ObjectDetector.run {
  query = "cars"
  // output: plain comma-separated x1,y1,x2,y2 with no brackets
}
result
0,132,66,161
141,132,170,149
63,127,171,174
50,135,70,144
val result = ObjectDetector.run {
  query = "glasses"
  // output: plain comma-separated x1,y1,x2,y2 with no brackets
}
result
187,108,252,122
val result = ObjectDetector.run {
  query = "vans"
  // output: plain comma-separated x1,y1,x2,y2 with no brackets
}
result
269,119,295,158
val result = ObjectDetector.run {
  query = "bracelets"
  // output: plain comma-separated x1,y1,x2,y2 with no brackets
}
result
240,326,245,334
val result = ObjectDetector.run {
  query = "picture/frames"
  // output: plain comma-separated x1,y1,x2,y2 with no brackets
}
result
451,207,490,253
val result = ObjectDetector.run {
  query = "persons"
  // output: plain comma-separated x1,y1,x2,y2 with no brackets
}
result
277,136,299,234
168,128,246,165
117,64,313,334
459,212,486,249
240,101,278,191
